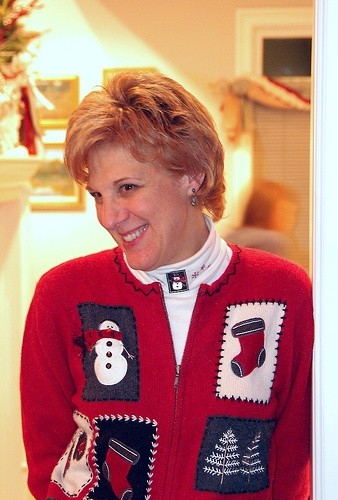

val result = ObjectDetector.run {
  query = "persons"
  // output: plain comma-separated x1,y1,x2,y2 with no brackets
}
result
21,68,311,500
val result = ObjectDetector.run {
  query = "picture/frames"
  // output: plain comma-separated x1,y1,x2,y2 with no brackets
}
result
102,67,156,86
29,158,83,211
35,76,80,129
